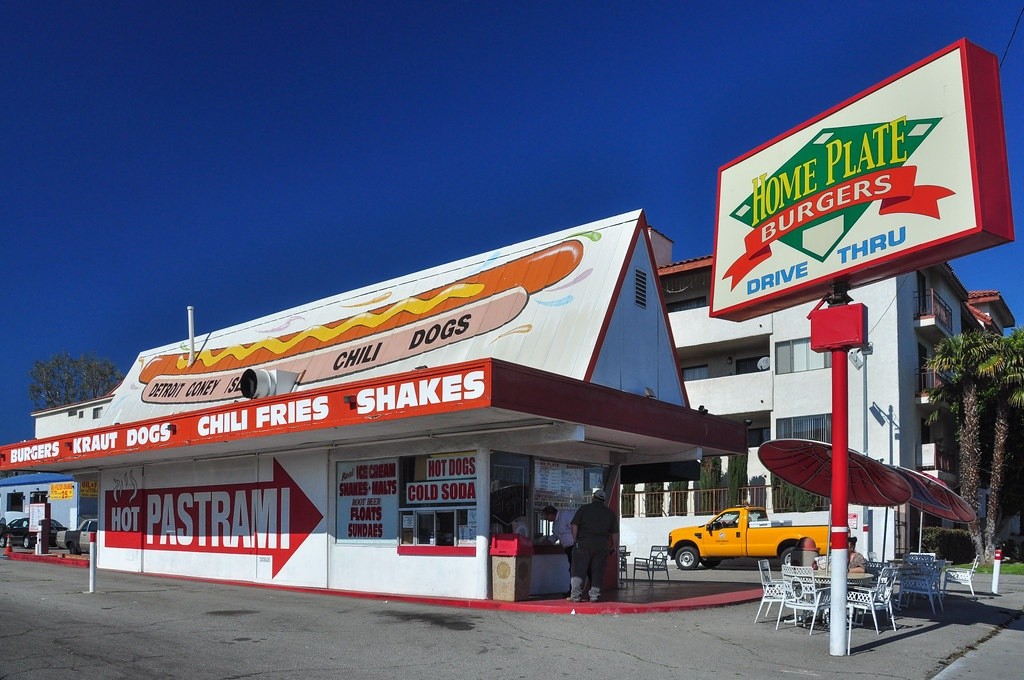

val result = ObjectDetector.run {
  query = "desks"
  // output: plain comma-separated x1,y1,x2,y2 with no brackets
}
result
886,558,953,599
793,570,874,626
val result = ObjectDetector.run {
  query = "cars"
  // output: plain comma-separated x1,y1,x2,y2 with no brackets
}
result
0,518,70,548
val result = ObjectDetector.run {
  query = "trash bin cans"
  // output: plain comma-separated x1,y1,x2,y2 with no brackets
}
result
489,533,533,601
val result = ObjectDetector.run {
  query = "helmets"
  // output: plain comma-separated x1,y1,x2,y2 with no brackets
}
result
593,490,605,499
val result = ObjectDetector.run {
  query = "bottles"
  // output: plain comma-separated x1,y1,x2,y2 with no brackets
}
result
429,532,434,545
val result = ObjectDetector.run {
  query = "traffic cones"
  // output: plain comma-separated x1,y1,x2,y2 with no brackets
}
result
3,535,14,556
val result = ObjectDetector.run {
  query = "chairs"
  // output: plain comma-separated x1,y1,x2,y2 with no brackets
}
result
618,546,630,589
942,554,980,600
753,552,947,655
632,545,671,589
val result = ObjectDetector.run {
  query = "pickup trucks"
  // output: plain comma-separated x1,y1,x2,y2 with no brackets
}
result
666,506,850,571
56,518,98,555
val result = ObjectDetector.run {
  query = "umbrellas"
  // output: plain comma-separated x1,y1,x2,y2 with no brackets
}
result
758,439,977,575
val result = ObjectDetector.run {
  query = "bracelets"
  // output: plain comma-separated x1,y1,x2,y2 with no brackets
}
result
848,568,849,573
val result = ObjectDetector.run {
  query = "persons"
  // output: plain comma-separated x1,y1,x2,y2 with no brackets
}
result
542,506,592,597
847,536,867,573
566,490,618,603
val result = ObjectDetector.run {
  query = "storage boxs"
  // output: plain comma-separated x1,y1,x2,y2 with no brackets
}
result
749,520,781,528
779,520,792,526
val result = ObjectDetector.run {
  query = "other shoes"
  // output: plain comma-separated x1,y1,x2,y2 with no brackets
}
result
566,596,597,602
